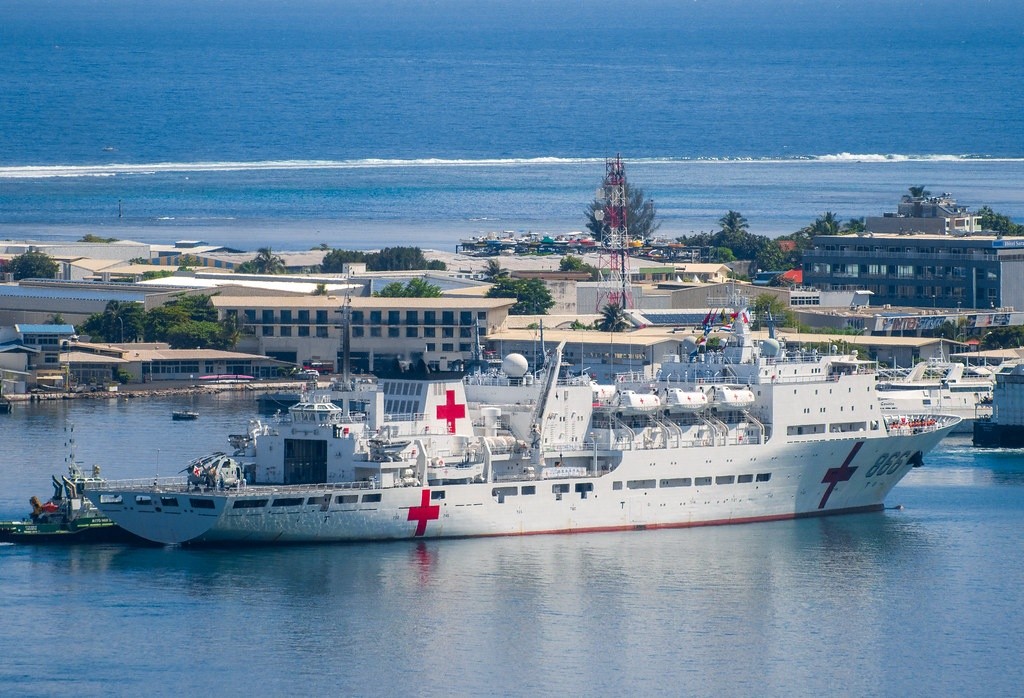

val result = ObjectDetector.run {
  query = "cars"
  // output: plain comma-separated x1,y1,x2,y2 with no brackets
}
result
306,370,319,379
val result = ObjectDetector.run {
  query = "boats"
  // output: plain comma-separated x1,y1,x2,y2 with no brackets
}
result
173,411,201,420
70,265,968,539
71,385,84,393
653,287,999,424
0,400,12,413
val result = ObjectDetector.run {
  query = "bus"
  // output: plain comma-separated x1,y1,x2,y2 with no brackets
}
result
302,360,334,375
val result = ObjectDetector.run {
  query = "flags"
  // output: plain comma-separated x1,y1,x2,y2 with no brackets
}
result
782,266,802,283
693,305,750,346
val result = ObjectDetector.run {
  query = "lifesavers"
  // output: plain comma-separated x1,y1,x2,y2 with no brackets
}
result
770,374,782,384
